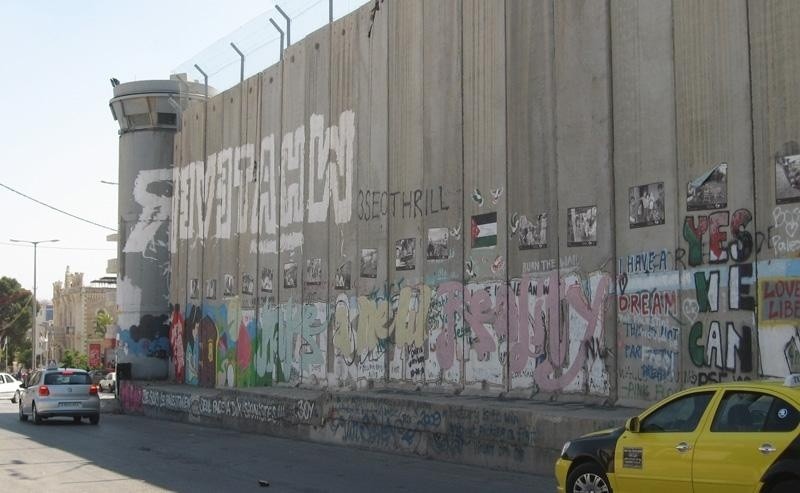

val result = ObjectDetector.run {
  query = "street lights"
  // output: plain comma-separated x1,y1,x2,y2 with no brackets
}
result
10,239,59,374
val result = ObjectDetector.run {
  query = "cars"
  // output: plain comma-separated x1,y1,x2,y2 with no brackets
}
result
19,368,103,425
554,379,800,493
0,372,26,403
98,372,116,392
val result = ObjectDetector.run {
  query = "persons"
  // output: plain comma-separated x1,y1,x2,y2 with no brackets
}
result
631,184,664,225
575,210,597,242
396,239,413,269
